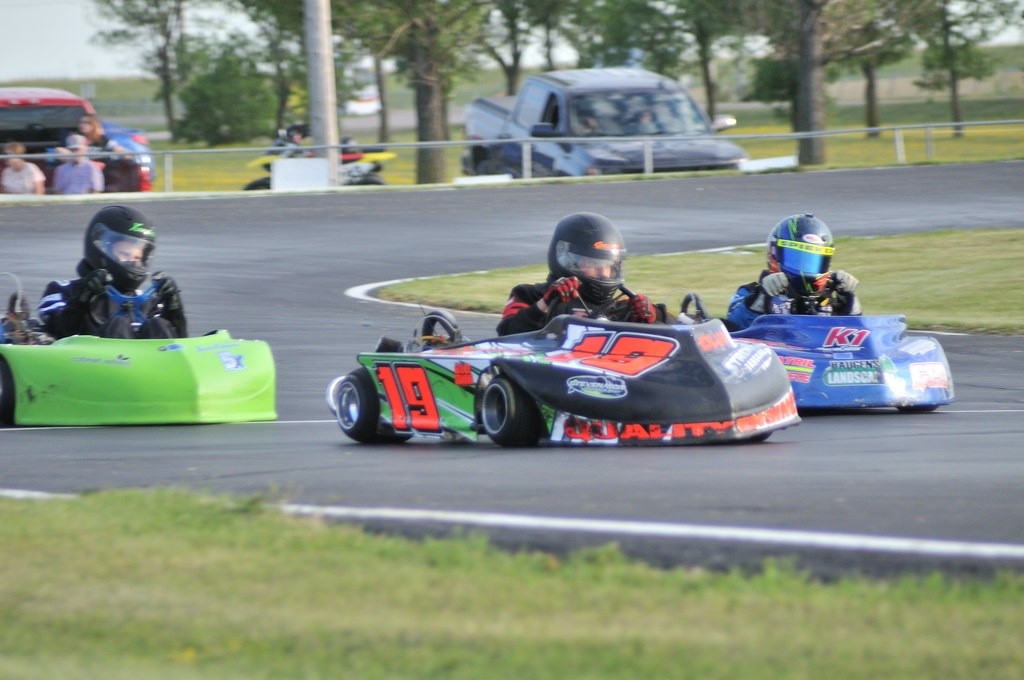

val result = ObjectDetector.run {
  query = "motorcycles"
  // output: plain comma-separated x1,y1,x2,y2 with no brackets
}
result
243,126,396,191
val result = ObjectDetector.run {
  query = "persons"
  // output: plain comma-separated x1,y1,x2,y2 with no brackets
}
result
54,114,123,170
39,205,187,338
53,135,105,193
495,212,679,336
727,213,861,331
2,142,46,194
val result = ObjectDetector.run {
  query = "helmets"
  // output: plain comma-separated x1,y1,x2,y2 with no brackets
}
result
547,211,626,304
83,204,157,293
766,213,835,295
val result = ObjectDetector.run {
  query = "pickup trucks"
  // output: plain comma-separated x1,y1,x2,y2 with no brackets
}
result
463,65,748,180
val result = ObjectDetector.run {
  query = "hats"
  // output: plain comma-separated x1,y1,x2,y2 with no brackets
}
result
66,135,86,149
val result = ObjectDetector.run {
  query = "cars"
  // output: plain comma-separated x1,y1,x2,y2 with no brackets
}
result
0,85,153,193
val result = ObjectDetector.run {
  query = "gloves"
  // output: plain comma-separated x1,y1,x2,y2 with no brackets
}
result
628,294,657,323
836,269,859,293
761,272,788,297
75,269,114,303
543,275,580,308
151,271,181,311
